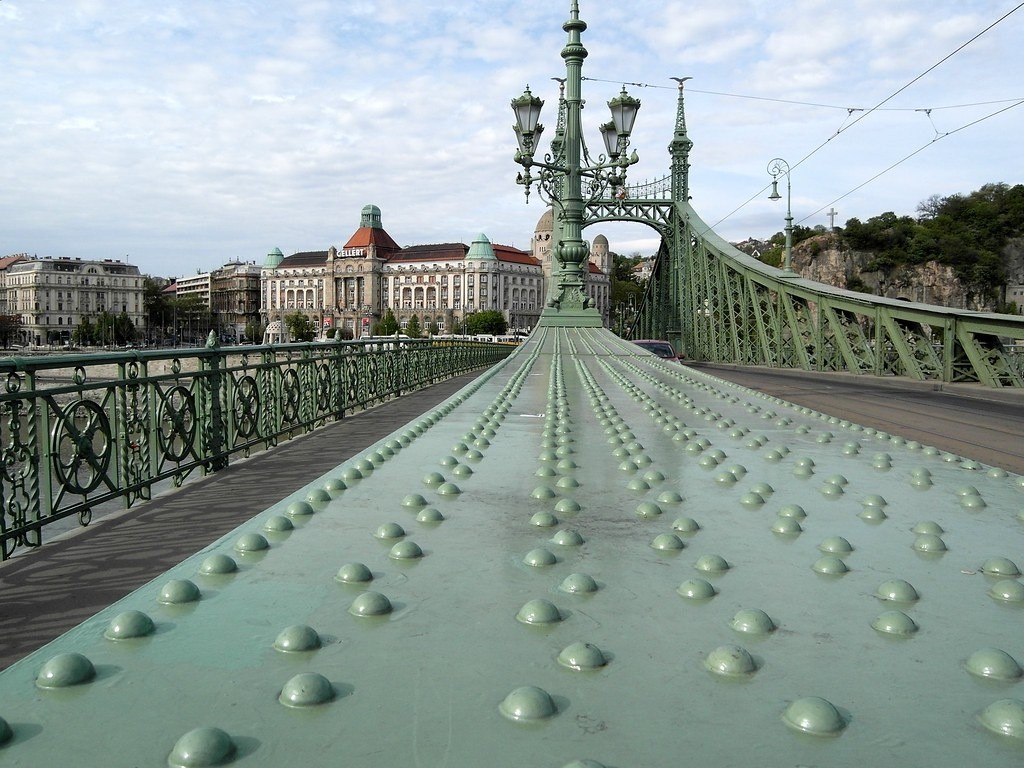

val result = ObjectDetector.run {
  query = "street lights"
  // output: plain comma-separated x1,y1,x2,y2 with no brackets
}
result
767,158,792,277
509,1,642,328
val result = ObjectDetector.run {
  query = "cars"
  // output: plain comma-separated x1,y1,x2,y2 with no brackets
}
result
631,339,685,365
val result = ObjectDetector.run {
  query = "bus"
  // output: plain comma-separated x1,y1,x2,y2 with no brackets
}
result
433,334,527,344
360,334,411,350
